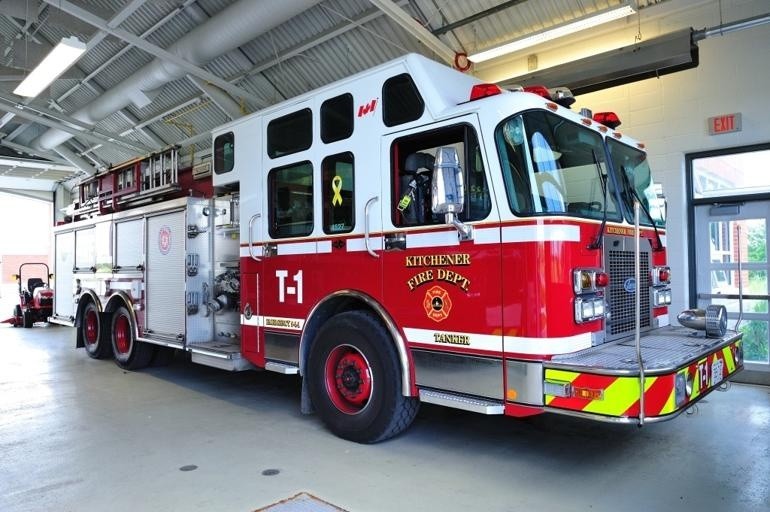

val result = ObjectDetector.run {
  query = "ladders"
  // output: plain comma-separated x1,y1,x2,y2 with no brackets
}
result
72,143,183,216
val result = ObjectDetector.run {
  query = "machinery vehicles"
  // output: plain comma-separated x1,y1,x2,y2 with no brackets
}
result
12,261,53,328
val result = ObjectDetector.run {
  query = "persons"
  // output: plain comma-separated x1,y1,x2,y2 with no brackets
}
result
407,167,439,223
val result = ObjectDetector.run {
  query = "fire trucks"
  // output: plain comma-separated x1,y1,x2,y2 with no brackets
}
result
45,52,748,443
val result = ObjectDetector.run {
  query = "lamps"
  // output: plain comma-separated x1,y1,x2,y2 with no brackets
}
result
464,0,640,66
12,0,86,99
